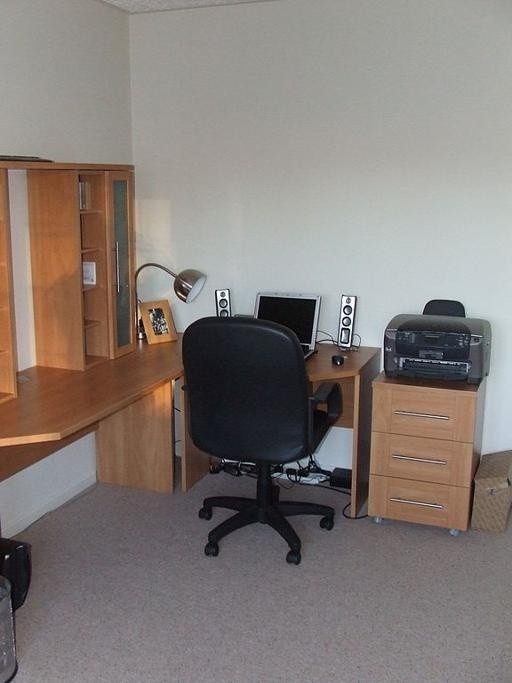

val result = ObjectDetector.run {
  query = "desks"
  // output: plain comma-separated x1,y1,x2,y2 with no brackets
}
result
0,334,380,519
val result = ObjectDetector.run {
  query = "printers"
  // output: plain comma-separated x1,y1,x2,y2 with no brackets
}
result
384,299,491,384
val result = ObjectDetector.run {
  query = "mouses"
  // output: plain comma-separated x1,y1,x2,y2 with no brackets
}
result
332,355,343,366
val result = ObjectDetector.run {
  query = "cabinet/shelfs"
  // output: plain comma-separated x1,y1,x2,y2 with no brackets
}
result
368,370,487,536
1,159,136,407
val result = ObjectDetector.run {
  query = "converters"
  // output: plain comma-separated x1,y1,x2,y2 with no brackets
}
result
330,467,351,489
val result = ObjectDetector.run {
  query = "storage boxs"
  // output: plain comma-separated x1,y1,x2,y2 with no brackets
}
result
471,449,512,531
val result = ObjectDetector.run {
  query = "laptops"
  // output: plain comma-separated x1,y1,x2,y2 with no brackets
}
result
254,293,321,358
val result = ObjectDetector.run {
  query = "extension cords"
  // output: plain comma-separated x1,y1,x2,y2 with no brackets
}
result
273,468,328,485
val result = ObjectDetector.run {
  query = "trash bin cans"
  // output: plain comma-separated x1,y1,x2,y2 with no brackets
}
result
0,575,18,683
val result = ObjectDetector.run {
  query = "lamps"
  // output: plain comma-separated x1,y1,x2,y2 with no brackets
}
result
135,263,207,339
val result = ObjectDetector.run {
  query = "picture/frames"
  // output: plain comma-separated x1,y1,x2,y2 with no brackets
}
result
139,299,179,346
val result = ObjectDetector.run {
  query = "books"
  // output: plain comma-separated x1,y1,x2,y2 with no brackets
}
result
79,182,88,211
82,262,97,285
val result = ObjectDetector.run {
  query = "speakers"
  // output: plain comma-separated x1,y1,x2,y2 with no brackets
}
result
338,295,357,348
215,289,231,318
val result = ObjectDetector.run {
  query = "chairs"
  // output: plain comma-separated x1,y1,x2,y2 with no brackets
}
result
182,316,335,564
